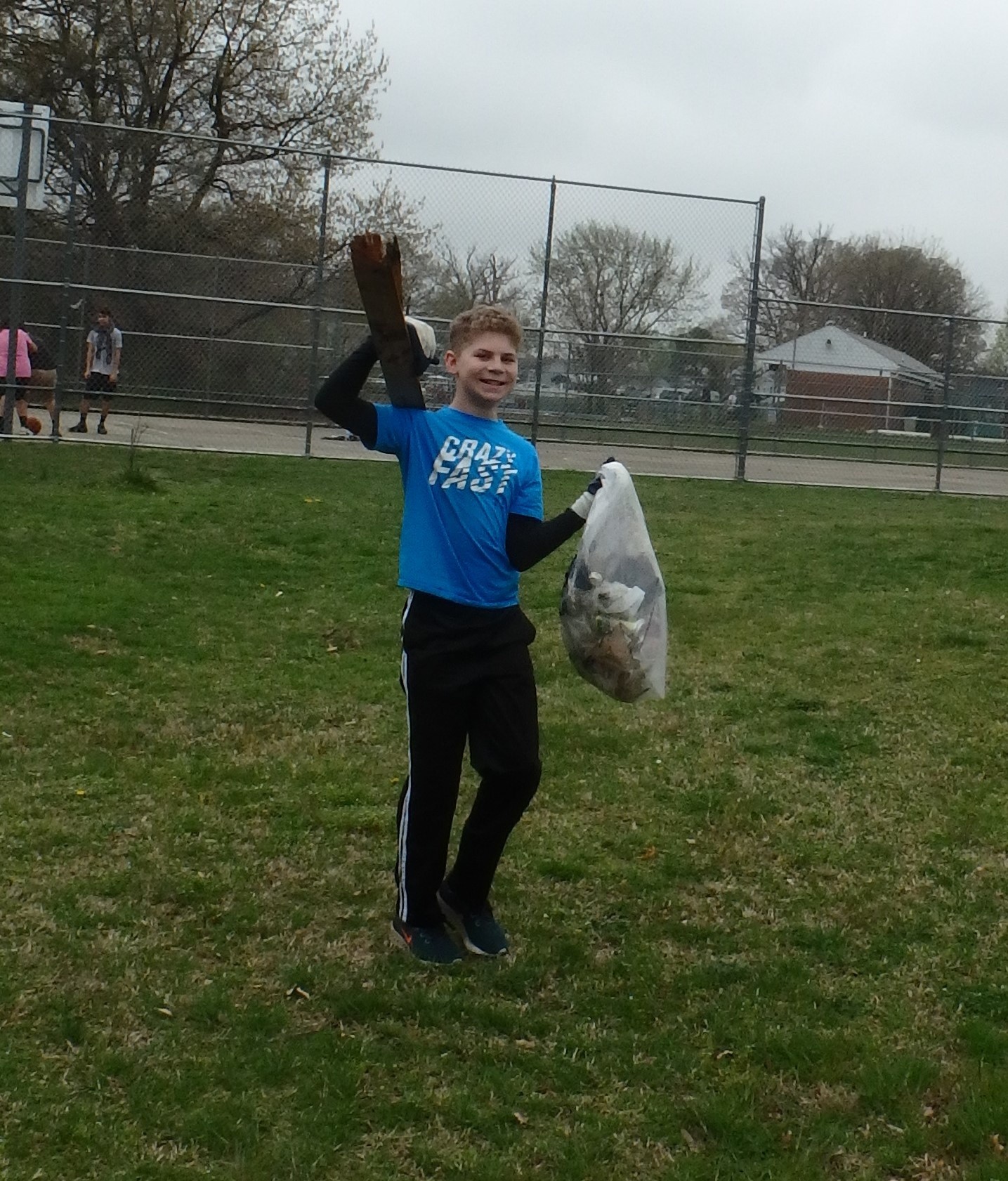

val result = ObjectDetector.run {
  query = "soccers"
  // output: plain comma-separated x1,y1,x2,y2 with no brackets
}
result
27,417,41,434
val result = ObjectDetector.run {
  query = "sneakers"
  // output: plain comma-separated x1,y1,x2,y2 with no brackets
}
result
390,917,462,966
98,424,107,434
69,422,87,432
437,881,509,957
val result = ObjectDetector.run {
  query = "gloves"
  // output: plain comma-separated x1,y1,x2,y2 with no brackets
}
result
588,457,620,496
405,315,440,376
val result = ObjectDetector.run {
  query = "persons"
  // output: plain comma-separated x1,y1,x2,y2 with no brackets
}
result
0,313,39,436
67,307,123,435
15,323,62,437
314,303,621,968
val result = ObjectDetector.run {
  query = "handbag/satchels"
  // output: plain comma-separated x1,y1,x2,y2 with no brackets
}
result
560,460,664,704
